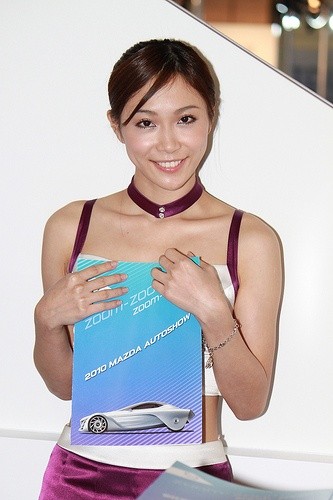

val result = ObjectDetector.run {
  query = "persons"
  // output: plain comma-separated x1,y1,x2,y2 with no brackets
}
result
33,37,283,500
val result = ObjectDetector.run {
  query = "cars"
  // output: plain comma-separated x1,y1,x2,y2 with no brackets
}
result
78,400,196,433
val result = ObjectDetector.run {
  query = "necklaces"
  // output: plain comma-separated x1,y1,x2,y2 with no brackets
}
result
127,175,204,219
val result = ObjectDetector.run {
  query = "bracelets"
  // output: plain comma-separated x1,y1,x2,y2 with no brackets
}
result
205,319,239,369
